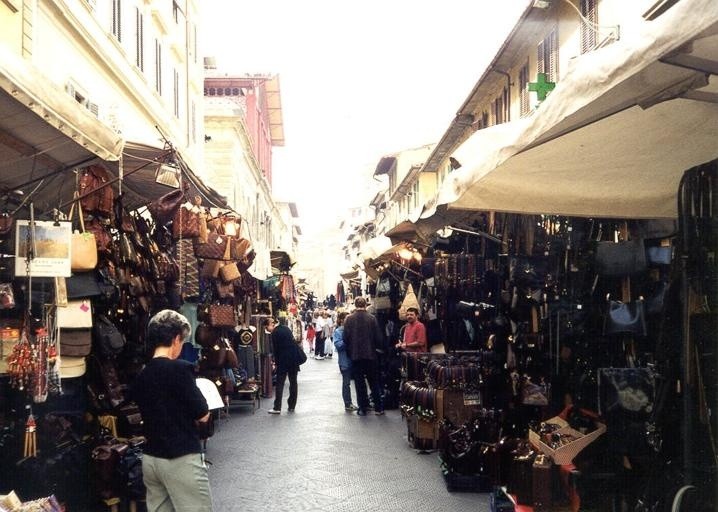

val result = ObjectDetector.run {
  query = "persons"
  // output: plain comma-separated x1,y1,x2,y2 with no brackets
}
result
395,306,429,380
333,311,356,411
263,316,308,414
220,336,236,419
299,300,338,362
340,295,385,414
365,304,401,411
133,308,217,511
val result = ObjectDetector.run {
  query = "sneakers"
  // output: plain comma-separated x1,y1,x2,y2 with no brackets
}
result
265,353,386,417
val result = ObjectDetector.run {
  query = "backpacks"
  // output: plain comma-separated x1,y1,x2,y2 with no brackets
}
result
436,419,490,495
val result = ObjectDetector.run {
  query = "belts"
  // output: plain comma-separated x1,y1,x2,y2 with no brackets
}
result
396,252,489,423
676,157,718,301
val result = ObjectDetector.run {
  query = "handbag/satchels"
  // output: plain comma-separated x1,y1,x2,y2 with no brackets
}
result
172,202,256,399
489,217,677,422
68,163,176,316
373,271,394,318
0,184,68,512
68,314,148,512
628,310,718,512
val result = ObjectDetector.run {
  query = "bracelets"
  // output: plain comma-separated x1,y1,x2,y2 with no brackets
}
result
405,343,408,348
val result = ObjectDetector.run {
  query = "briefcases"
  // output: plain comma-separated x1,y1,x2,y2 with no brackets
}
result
487,434,560,512
